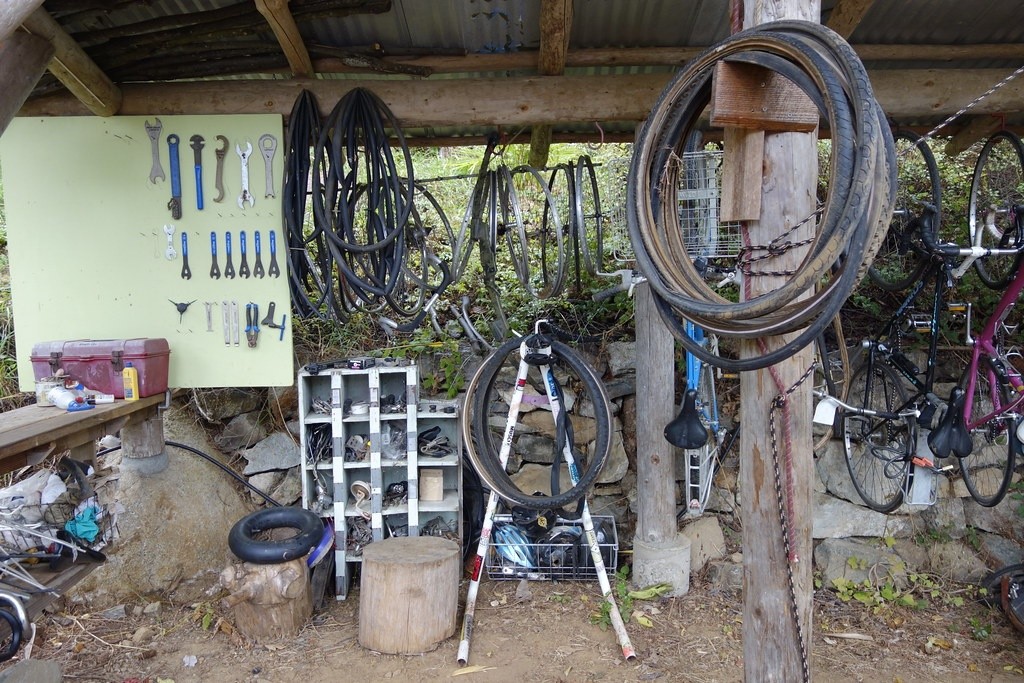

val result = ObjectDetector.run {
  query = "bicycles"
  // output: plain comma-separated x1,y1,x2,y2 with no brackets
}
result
842,127,1024,515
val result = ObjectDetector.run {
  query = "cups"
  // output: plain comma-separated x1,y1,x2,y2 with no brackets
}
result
34,378,65,407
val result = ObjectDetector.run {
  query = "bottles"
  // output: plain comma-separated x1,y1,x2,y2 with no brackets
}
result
76,393,114,405
47,386,77,410
122,361,139,401
68,380,90,392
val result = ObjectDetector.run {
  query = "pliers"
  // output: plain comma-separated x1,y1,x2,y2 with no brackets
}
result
245,304,260,348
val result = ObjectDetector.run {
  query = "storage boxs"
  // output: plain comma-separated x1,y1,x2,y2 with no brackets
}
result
30,339,170,397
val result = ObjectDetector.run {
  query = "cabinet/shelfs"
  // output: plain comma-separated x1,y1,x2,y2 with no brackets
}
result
300,363,462,599
